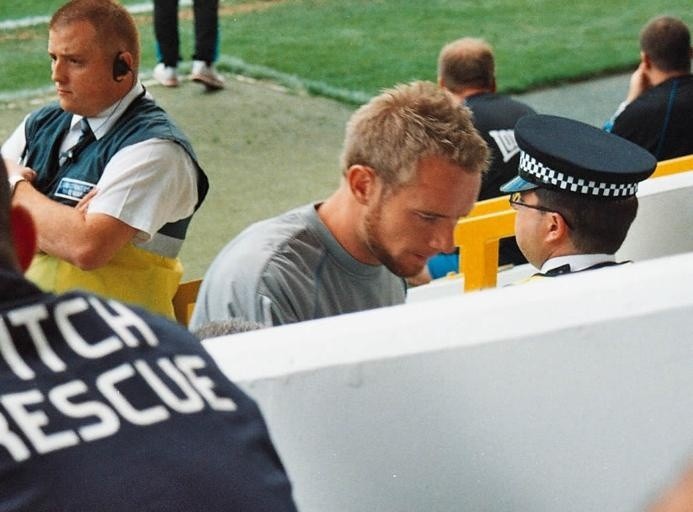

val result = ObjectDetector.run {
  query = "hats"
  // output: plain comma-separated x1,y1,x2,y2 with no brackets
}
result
500,115,657,200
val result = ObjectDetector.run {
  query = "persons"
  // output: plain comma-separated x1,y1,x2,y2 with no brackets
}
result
0,156,298,511
0,0,209,323
603,16,693,161
188,79,491,339
496,112,658,286
151,0,228,88
408,38,538,288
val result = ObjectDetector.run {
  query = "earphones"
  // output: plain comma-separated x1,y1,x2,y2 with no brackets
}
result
113,50,130,83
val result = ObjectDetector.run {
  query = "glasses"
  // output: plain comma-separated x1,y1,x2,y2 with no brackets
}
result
508,192,572,229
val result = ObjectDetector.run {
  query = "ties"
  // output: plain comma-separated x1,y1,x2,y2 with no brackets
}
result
75,119,90,144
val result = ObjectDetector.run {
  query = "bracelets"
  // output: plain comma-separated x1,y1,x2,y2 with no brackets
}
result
8,173,25,198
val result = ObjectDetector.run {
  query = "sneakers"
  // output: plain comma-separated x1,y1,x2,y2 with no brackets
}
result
152,63,178,85
189,59,223,88
